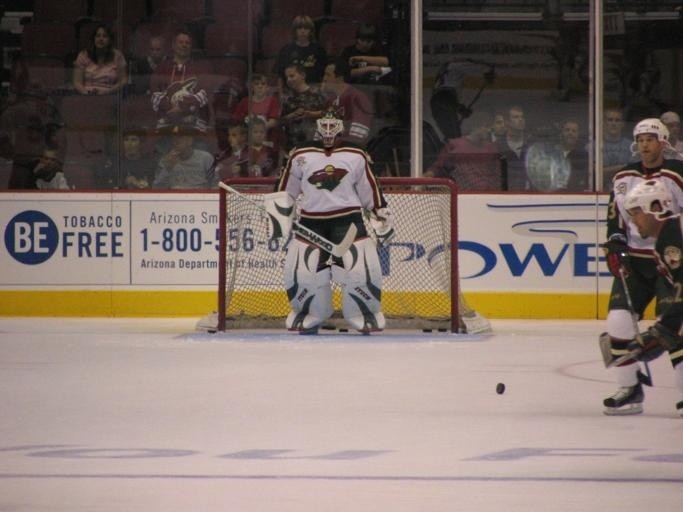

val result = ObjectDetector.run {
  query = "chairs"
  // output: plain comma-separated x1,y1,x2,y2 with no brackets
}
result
0,0,407,181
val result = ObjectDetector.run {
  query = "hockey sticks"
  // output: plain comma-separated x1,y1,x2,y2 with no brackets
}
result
621,268,654,386
218,180,358,257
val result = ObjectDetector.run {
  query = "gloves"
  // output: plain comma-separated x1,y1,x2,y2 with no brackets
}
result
604,239,631,277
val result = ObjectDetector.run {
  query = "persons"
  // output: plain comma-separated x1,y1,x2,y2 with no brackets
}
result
266,107,394,337
1,1,682,191
603,119,683,415
624,179,682,359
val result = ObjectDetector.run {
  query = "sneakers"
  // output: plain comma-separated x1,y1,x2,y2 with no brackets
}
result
604,381,643,408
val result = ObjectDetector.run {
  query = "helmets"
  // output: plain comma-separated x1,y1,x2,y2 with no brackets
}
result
633,118,669,145
622,179,680,214
